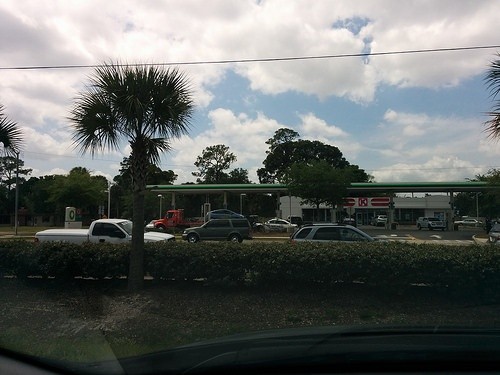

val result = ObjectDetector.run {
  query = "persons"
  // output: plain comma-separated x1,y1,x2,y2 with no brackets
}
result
485,219,492,234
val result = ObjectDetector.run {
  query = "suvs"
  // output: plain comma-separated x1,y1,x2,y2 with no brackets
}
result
370,215,387,226
416,217,446,230
290,223,374,244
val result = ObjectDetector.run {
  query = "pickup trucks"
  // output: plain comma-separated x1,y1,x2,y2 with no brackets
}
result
34,219,177,248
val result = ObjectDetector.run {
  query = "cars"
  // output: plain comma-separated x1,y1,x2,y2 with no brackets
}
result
344,216,354,222
453,219,483,226
482,220,500,240
182,210,255,244
249,215,304,229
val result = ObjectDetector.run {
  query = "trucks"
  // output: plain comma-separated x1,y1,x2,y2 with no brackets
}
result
146,209,203,231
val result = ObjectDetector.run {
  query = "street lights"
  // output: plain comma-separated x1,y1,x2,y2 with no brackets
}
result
105,179,119,218
471,192,483,215
240,193,247,213
158,194,164,220
